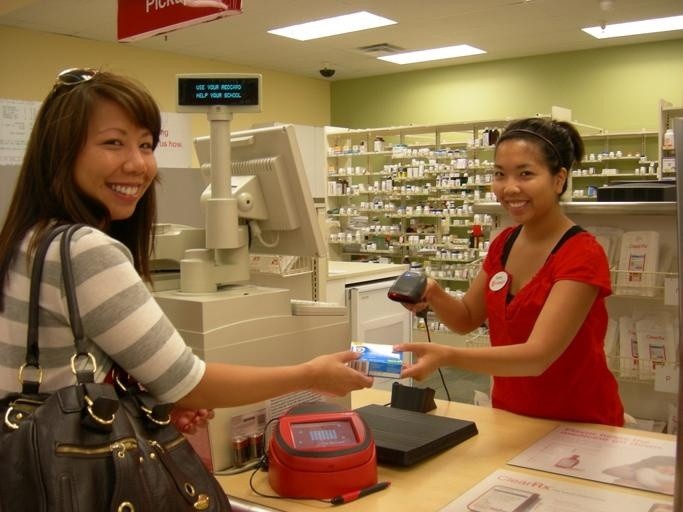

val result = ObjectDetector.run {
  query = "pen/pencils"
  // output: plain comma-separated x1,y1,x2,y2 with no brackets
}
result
332,481,391,504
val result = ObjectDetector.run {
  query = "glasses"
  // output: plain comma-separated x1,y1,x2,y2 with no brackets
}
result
52,66,103,88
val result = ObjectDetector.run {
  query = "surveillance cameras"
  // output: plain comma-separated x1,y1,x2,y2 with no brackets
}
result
320,67,335,82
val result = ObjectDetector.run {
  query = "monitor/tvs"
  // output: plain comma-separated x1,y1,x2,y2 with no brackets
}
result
194,123,327,257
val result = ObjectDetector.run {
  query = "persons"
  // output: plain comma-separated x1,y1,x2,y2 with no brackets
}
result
2,67,374,509
391,118,626,429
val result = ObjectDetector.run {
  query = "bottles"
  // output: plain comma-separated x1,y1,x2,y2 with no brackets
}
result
663,129,674,149
326,125,499,278
570,150,654,199
417,288,466,332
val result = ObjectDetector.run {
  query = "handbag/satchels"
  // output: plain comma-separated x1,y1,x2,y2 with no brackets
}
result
0,375,233,512
